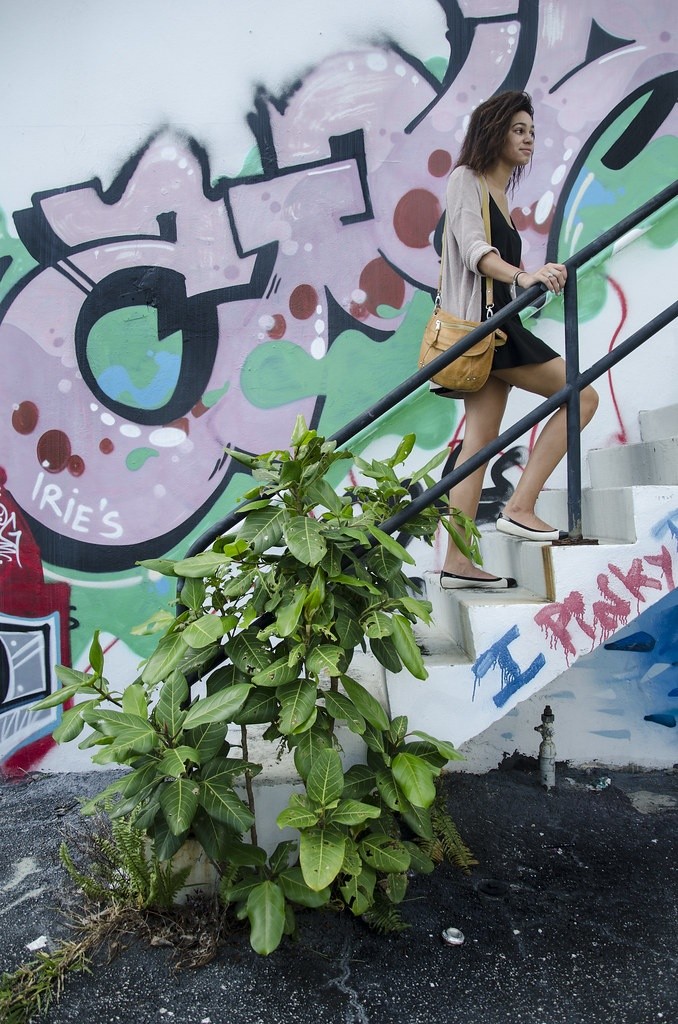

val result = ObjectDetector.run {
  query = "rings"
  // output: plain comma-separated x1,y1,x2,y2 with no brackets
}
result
545,273,553,278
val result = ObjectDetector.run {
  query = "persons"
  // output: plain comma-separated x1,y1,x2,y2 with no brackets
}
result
419,94,600,590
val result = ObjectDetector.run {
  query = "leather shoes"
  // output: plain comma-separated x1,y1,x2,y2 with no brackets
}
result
495,512,568,541
440,570,517,589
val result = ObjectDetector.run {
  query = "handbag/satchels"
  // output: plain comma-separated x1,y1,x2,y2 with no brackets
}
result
418,308,507,392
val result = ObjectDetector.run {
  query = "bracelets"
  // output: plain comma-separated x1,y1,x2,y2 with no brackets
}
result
512,270,528,286
